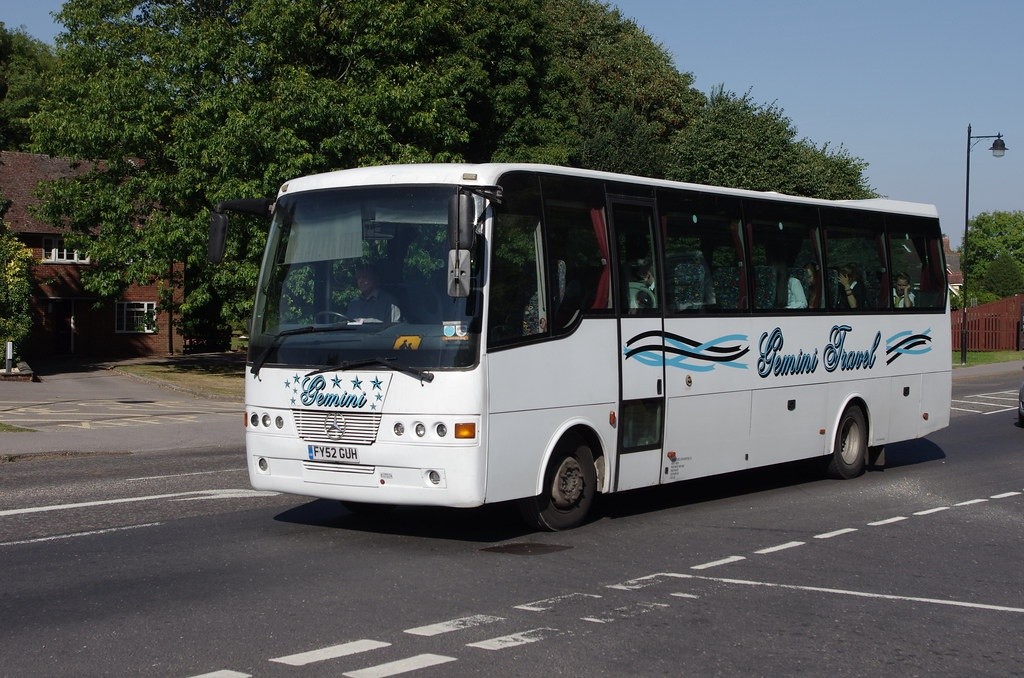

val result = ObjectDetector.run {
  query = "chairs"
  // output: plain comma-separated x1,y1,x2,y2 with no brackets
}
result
500,259,883,341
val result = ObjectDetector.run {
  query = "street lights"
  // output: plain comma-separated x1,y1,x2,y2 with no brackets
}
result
961,134,1008,365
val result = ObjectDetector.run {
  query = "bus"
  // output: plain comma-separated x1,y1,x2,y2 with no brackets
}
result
239,163,953,531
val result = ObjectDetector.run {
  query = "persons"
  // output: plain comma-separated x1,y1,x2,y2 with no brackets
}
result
833,262,865,308
785,271,808,309
338,263,391,324
893,272,915,307
637,265,678,309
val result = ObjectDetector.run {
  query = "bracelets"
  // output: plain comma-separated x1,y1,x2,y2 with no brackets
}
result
847,293,853,296
845,289,851,291
